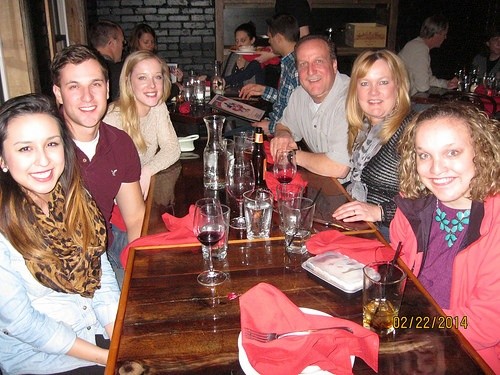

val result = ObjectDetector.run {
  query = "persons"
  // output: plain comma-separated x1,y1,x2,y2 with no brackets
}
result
239,13,299,138
471,31,500,88
0,93,121,375
128,23,158,54
49,44,146,292
396,15,460,100
219,21,263,89
254,0,311,64
331,47,418,244
269,34,353,185
388,100,500,375
88,19,183,104
102,50,181,202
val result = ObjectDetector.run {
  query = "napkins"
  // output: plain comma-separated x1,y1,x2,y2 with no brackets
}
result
266,169,307,202
263,139,276,164
239,283,380,375
119,205,226,270
306,230,404,267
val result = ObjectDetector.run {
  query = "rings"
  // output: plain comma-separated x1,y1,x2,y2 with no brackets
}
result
354,210,356,216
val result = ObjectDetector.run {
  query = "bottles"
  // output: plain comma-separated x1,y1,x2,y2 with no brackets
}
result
210,60,223,97
251,127,267,185
202,115,229,190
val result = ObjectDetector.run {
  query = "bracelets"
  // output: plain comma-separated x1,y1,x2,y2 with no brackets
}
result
379,206,383,224
287,149,299,164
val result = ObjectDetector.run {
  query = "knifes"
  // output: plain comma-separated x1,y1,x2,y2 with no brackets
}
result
313,218,352,231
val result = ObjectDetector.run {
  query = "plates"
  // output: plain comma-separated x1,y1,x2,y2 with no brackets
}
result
238,307,355,375
230,50,257,55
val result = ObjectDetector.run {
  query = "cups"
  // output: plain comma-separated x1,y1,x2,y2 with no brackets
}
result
454,64,497,93
223,132,253,184
282,197,316,255
363,262,408,337
198,204,231,261
243,189,273,241
181,69,210,106
275,183,304,233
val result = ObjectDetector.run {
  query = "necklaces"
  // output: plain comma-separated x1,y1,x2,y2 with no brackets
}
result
436,199,441,217
32,191,52,209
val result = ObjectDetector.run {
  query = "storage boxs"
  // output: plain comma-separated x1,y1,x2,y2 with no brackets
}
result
346,22,387,48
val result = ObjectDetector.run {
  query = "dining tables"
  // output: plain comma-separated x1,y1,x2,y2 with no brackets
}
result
104,79,500,375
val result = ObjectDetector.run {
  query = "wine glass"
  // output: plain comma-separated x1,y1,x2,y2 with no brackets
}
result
273,148,297,214
225,158,256,230
192,199,227,287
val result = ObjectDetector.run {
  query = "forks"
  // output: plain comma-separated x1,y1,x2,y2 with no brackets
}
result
244,325,352,342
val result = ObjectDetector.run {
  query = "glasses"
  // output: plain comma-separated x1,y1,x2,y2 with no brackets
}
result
108,38,126,47
